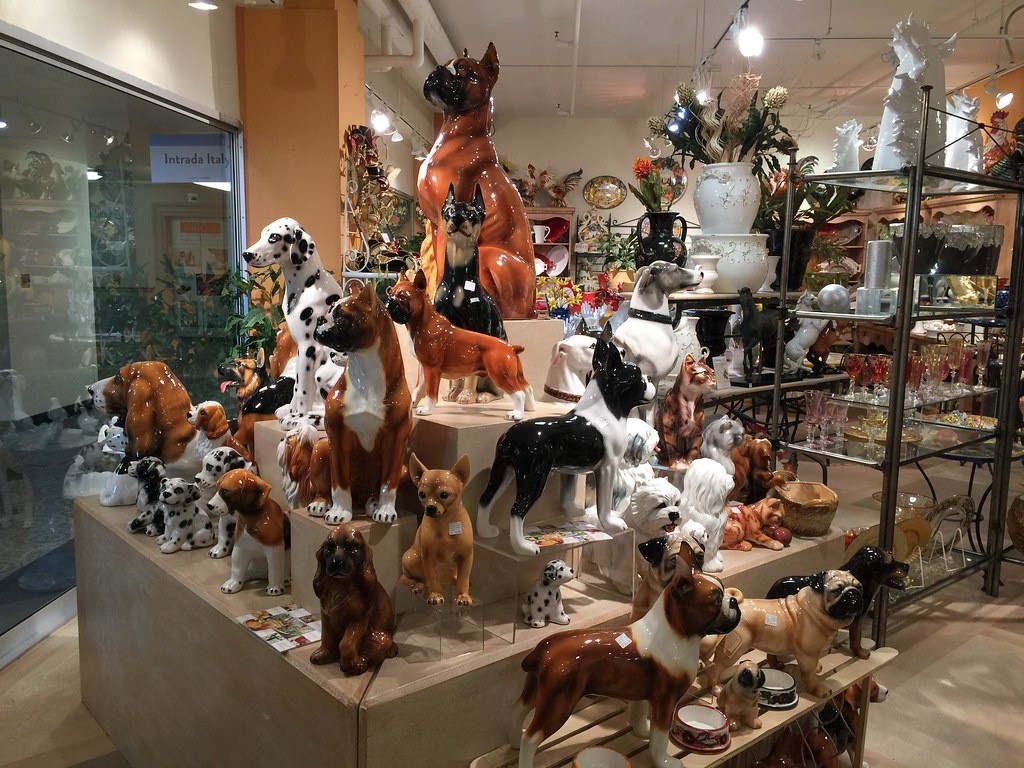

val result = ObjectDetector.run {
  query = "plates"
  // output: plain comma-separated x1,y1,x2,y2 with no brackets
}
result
843,518,932,581
534,258,547,275
545,246,569,277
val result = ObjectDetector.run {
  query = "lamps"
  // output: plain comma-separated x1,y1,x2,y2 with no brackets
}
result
983,73,1014,110
370,99,391,132
391,112,404,143
409,133,422,155
60,119,79,144
20,109,43,136
103,129,115,147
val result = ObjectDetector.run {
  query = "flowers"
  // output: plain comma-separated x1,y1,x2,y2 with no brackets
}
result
95,253,233,402
535,276,583,308
219,264,283,356
628,156,664,213
645,73,798,183
752,155,858,232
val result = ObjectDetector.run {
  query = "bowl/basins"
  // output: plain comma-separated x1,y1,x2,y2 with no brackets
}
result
758,669,799,710
573,746,631,768
687,234,769,294
670,704,731,751
775,482,839,538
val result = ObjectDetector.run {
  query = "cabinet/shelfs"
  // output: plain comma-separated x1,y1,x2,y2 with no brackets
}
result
524,206,576,286
0,145,94,323
762,84,1024,650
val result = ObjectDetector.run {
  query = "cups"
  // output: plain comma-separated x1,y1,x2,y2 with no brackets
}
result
533,225,550,244
692,257,718,293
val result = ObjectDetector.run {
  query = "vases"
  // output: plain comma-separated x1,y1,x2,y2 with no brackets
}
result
635,212,688,270
691,255,721,293
551,308,570,322
760,229,815,292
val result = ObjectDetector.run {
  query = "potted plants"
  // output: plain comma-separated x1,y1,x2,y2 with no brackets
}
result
806,229,851,311
594,228,638,291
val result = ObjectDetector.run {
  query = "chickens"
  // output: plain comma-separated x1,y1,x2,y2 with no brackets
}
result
982,110,1024,184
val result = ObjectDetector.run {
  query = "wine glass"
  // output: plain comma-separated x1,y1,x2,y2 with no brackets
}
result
907,338,990,398
803,391,848,449
856,414,888,460
842,354,892,403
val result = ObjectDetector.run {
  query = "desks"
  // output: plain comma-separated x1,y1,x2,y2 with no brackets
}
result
469,629,899,768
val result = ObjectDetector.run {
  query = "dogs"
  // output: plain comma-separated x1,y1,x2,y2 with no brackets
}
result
81,40,910,768
